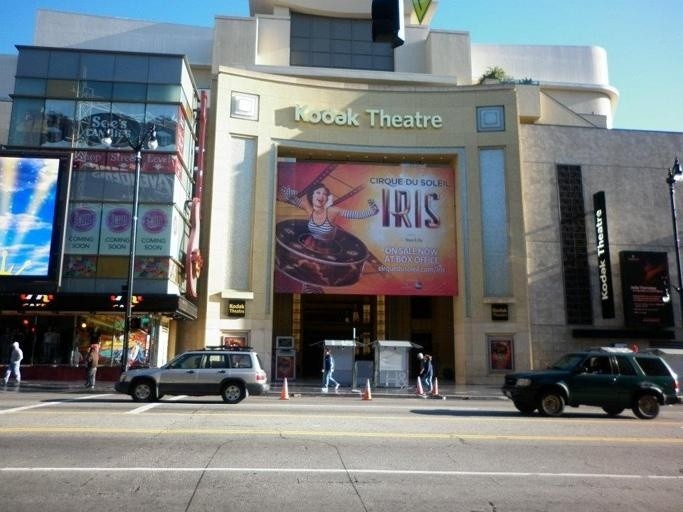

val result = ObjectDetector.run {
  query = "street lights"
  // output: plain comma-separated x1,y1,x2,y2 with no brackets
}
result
100,122,159,374
659,157,683,333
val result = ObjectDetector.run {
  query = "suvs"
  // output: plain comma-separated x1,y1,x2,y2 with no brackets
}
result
115,345,270,404
501,347,682,420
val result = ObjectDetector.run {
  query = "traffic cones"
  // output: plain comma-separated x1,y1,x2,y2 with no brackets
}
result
416,376,443,399
362,378,372,401
278,377,290,400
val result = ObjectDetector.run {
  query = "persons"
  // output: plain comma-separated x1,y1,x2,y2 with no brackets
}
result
85,346,98,389
423,353,434,393
415,352,425,388
2,340,24,385
277,182,380,241
1,320,148,367
84,345,91,387
320,347,340,392
